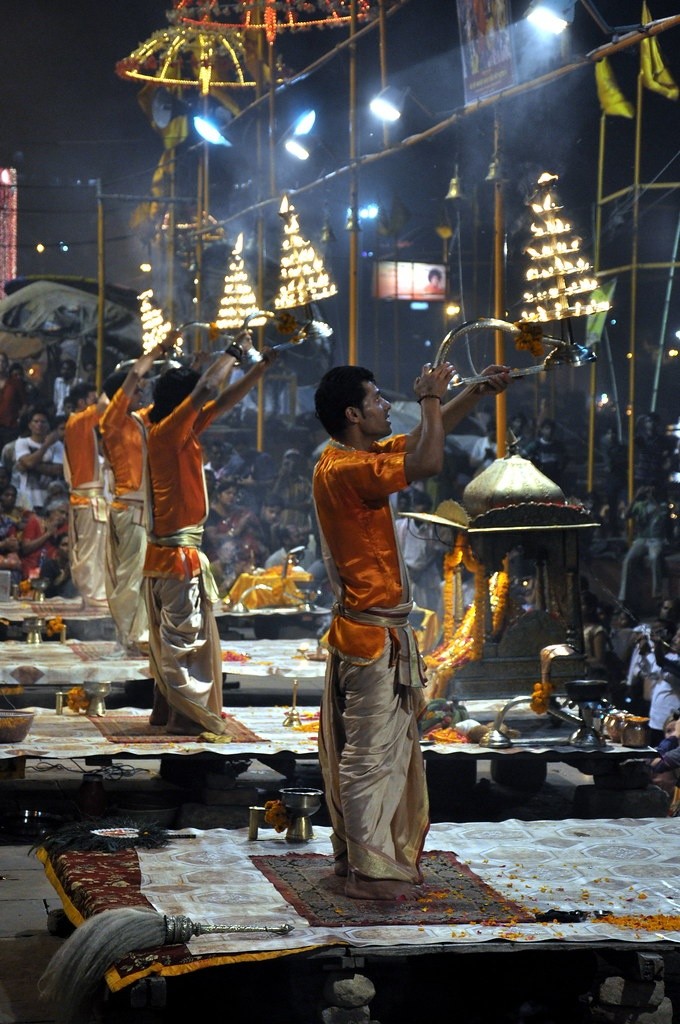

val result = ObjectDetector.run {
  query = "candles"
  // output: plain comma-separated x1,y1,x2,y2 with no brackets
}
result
515,170,614,324
135,290,185,361
273,195,339,311
214,232,269,330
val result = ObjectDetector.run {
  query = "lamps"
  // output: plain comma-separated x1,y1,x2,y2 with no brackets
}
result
193,115,235,148
522,0,645,35
367,83,440,124
284,139,328,182
274,108,316,137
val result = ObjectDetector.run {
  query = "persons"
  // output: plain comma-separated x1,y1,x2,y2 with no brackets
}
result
0,249,680,912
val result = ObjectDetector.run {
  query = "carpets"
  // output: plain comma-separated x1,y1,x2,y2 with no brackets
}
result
30,601,110,617
66,636,252,662
84,710,269,744
245,850,536,929
